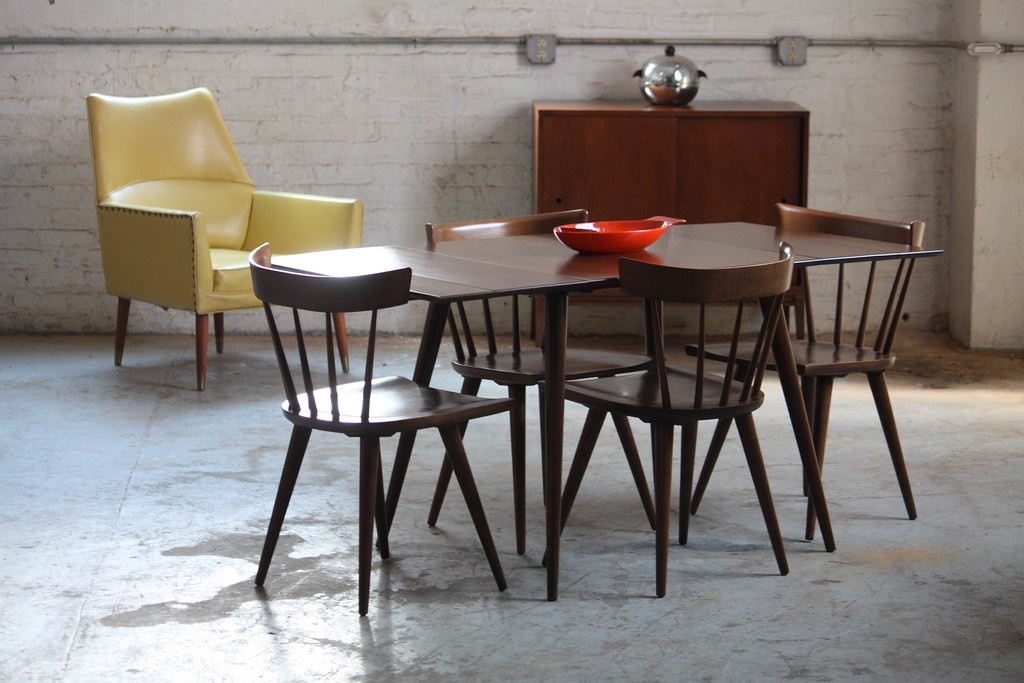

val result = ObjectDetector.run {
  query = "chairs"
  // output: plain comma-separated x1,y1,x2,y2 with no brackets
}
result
537,242,794,602
84,87,364,392
248,242,517,615
690,202,926,552
424,208,655,557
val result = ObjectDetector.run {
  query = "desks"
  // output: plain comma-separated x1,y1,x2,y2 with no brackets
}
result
269,222,943,603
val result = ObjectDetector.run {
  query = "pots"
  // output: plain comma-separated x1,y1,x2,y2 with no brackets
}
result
633,46,708,106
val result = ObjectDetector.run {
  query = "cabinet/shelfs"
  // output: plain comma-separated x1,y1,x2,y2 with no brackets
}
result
531,99,811,349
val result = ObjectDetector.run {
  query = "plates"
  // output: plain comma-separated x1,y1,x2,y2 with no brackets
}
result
553,214,686,254
